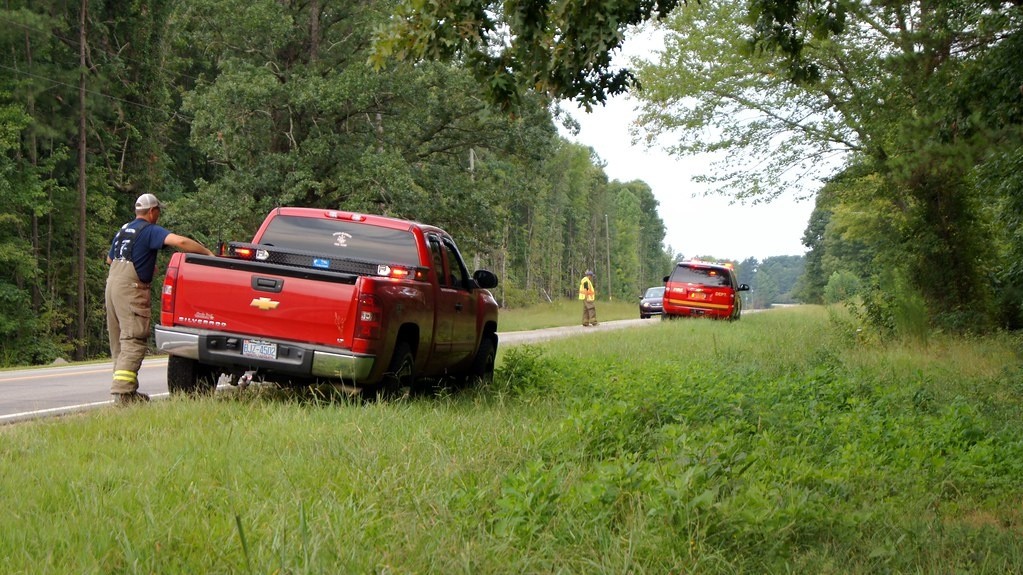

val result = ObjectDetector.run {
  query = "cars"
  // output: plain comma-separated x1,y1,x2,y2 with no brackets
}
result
639,287,666,319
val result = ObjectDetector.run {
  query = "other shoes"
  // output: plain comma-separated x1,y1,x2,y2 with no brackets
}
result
114,390,151,407
593,323,601,326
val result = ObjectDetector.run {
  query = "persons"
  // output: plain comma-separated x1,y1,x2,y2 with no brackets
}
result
105,194,214,409
579,271,600,327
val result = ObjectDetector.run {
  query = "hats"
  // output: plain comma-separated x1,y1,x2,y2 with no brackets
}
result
135,193,167,210
586,271,594,276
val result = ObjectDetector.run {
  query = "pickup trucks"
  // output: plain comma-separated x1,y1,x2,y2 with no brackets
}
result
660,258,749,322
152,206,501,407
